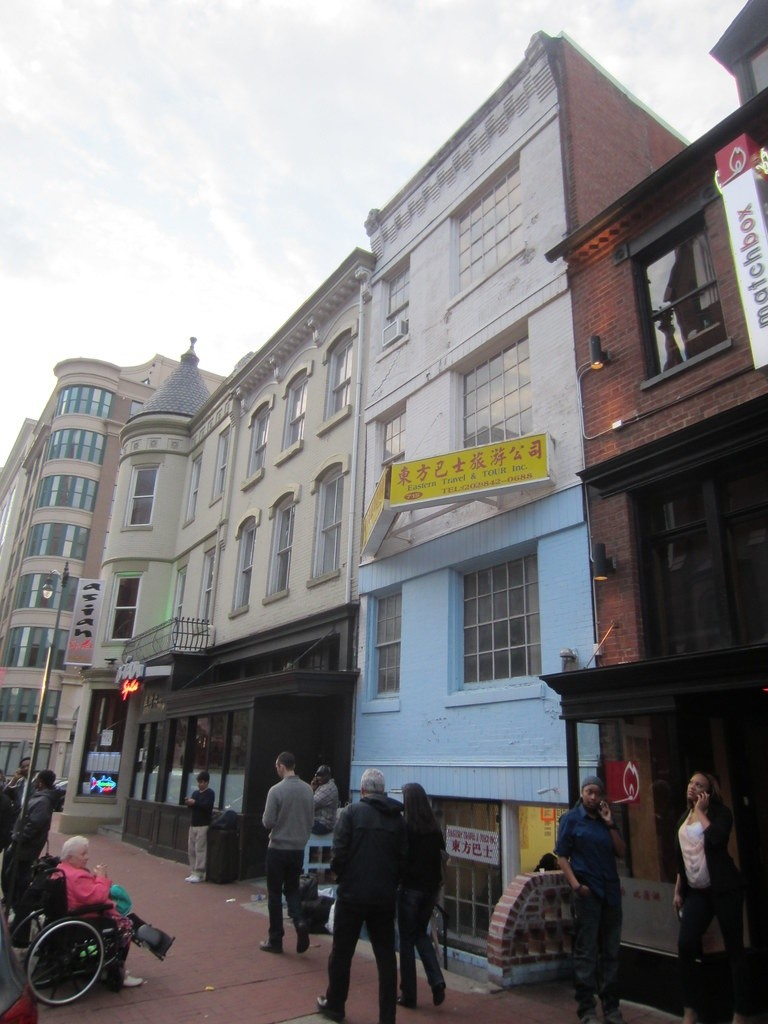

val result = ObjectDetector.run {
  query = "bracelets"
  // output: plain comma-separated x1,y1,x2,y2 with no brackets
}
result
606,823,619,831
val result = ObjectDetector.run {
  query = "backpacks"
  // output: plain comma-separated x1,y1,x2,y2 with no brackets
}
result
0,798,22,853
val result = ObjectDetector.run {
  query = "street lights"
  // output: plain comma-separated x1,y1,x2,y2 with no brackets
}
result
18,569,65,829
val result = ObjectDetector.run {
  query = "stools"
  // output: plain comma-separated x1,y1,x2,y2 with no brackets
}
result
302,833,334,876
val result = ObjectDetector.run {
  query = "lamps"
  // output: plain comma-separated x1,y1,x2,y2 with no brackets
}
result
589,335,604,369
593,542,608,581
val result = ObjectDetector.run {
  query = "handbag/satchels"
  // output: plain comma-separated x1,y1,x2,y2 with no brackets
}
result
439,848,450,887
300,872,337,933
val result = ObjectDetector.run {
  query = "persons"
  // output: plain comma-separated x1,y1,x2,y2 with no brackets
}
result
316,768,409,1024
50,834,144,988
396,783,446,1010
311,764,339,835
258,751,314,954
0,757,60,904
672,772,753,1024
553,775,627,1024
183,771,215,884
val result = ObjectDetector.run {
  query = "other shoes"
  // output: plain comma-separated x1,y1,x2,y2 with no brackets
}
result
432,982,445,1005
259,940,283,953
395,996,416,1009
296,920,310,953
315,995,345,1022
101,969,131,982
184,873,204,883
604,1010,628,1024
580,1010,603,1024
123,976,144,988
1,896,8,905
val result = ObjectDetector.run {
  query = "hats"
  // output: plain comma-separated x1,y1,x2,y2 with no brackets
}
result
580,775,605,797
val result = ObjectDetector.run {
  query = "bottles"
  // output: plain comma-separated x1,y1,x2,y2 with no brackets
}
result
251,894,266,901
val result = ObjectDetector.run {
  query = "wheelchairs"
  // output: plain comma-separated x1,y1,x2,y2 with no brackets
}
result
8,857,133,1008
